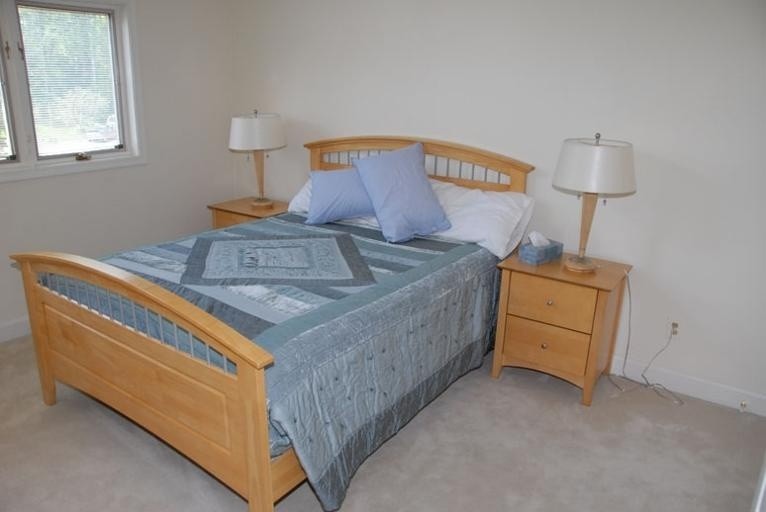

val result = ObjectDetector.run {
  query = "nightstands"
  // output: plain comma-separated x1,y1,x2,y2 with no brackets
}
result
205,195,290,231
490,250,633,407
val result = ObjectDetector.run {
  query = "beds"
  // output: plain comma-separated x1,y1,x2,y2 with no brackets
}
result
7,135,536,512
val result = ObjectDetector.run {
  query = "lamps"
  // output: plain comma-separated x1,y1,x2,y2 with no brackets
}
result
551,132,638,275
226,107,288,210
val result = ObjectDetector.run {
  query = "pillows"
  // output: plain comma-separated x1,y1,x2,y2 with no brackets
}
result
287,174,536,262
350,142,453,243
304,167,375,225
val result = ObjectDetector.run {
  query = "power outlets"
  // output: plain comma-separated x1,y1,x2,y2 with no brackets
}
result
666,318,681,341
